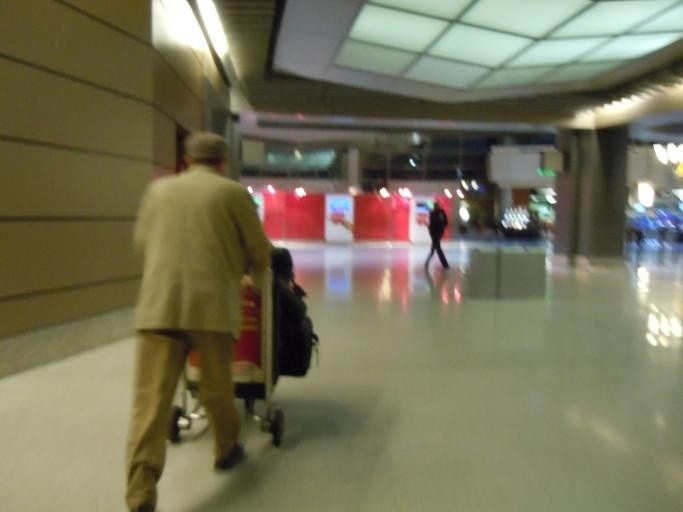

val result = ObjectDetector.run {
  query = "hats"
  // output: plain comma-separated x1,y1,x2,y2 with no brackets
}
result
182,128,227,162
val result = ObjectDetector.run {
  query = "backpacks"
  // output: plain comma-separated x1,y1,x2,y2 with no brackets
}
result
272,246,320,379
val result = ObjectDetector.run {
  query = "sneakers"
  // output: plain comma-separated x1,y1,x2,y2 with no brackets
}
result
210,444,246,475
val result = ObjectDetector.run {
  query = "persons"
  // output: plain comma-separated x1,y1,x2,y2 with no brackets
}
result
421,199,451,272
120,127,277,510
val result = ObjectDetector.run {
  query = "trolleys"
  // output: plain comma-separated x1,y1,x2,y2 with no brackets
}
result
167,248,320,448
